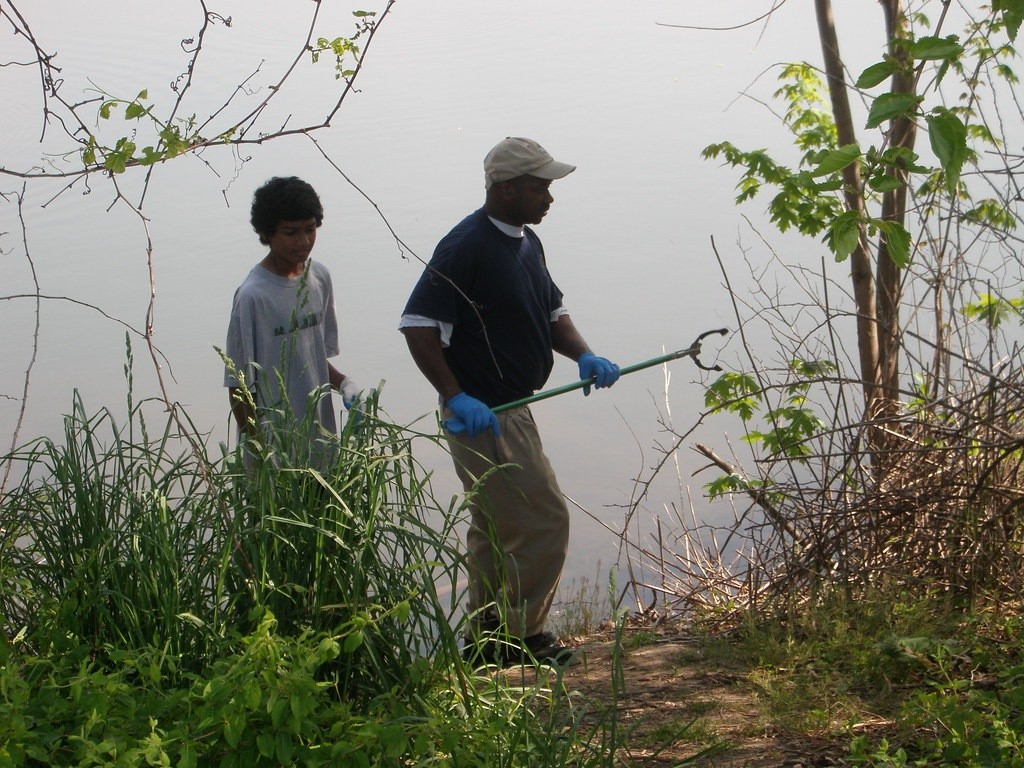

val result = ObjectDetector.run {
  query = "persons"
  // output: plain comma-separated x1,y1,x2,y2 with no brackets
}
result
226,175,369,523
399,136,620,665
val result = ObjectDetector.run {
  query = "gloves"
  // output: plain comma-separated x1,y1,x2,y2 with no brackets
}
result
339,377,371,434
579,352,620,396
447,393,501,439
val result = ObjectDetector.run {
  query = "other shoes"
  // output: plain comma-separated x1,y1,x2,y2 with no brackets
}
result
507,634,581,667
460,637,506,665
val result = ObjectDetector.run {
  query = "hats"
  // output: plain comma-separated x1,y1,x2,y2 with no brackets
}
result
482,137,577,189
